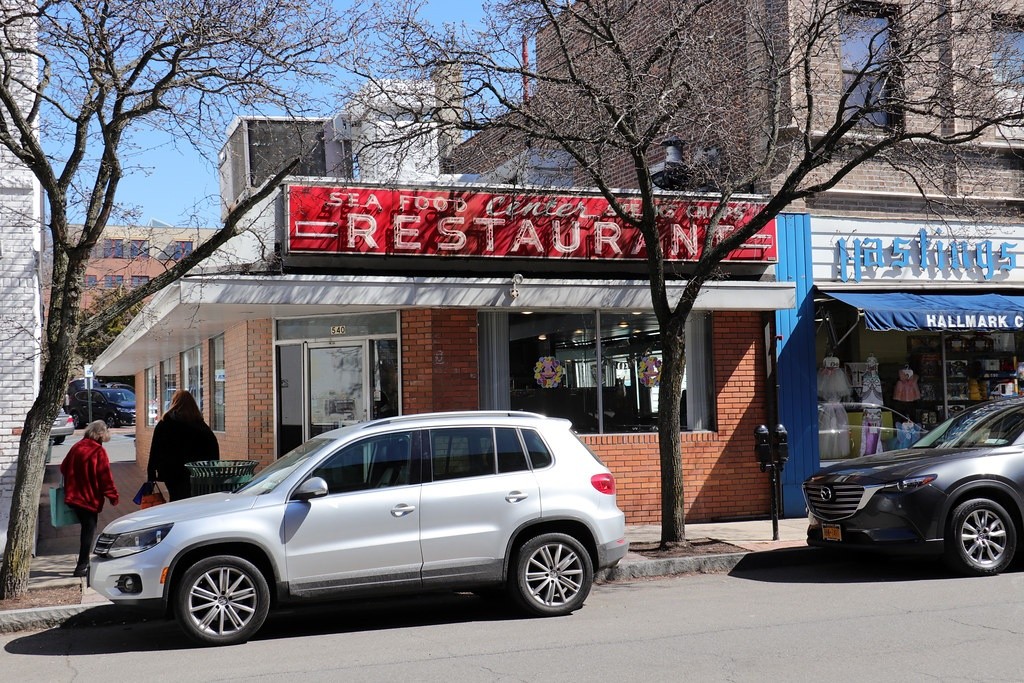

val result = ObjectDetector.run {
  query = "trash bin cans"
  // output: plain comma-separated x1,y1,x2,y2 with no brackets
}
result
184,458,260,499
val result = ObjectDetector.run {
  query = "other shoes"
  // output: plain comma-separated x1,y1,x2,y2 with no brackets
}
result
73,564,88,577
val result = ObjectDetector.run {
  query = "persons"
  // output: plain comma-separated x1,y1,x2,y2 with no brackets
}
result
147,390,219,503
61,419,119,577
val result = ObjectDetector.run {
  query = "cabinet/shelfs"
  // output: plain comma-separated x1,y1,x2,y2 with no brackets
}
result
907,329,1024,432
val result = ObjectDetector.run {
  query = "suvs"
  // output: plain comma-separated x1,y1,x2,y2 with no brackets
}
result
84,408,628,646
801,396,1024,576
68,389,138,429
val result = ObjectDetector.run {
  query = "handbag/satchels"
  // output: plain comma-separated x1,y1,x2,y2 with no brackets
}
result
49,485,80,527
140,492,167,510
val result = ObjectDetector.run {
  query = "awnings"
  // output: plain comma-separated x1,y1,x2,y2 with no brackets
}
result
825,292,1024,334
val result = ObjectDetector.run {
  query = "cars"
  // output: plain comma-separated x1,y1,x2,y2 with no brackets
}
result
46,407,75,446
